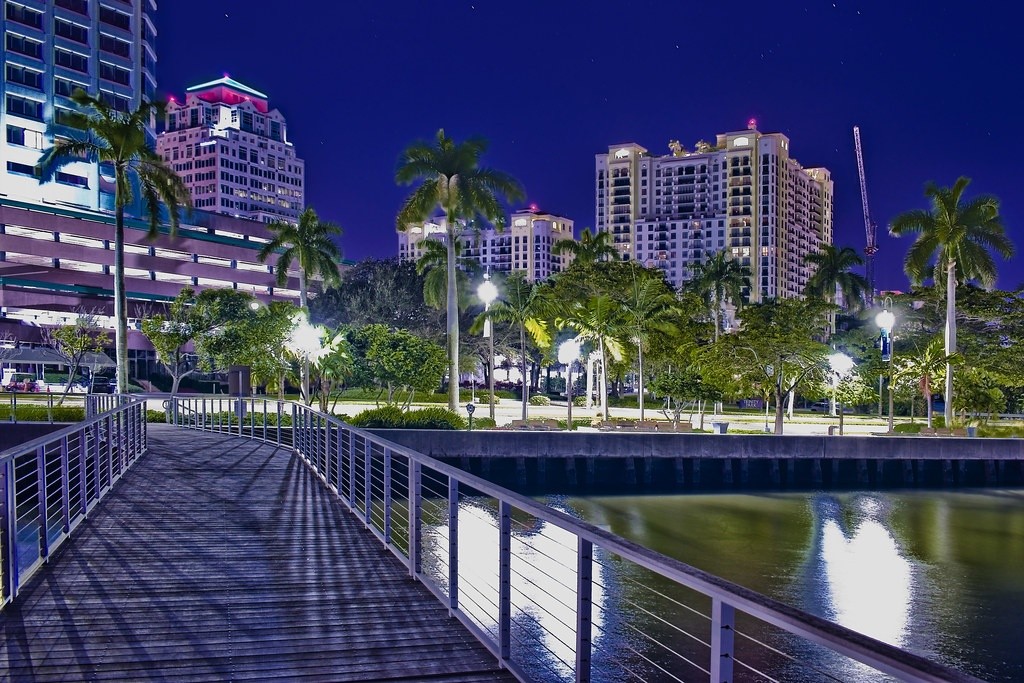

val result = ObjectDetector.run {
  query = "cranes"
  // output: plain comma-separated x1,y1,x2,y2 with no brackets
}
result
853,126,879,307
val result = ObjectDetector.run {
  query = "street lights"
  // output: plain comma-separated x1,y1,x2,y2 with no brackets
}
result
874,296,895,433
476,273,498,420
630,336,645,422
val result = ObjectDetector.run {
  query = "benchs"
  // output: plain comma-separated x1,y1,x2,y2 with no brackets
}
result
919,426,968,437
602,421,692,432
512,420,563,430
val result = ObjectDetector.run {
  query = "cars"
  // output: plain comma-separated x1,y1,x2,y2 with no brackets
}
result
606,382,634,397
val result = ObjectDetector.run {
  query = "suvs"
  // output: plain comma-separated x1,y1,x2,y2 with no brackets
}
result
5,372,36,392
87,377,114,395
